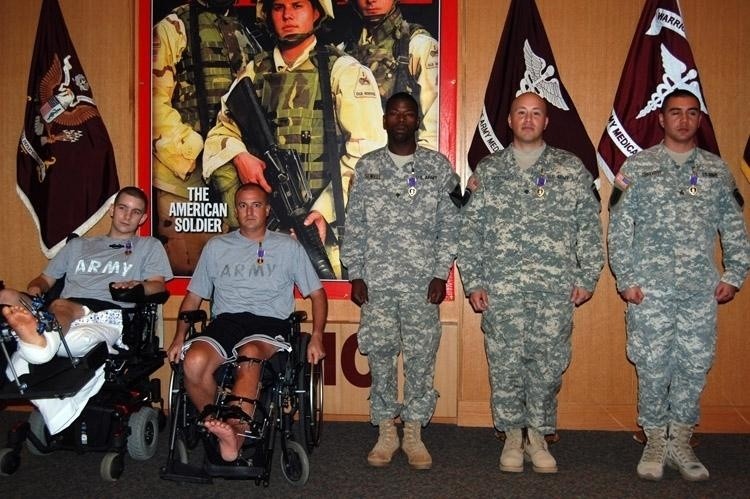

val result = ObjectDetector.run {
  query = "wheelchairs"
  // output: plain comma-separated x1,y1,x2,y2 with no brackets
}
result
0,234,169,482
160,309,323,487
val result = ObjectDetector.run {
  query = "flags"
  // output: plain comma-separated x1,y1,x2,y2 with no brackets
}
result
15,0,120,261
596,1,743,211
467,1,601,204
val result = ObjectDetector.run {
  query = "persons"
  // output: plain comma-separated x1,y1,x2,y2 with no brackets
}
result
457,92,604,475
338,92,463,469
606,91,750,482
202,0,388,278
0,187,173,365
154,1,262,275
167,183,327,461
335,1,439,150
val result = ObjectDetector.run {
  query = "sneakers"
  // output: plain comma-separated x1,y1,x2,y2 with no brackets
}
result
637,427,667,482
523,427,558,474
400,420,433,471
667,420,711,483
366,418,401,468
498,427,525,474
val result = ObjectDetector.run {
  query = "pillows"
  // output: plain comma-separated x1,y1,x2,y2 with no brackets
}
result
5,322,123,382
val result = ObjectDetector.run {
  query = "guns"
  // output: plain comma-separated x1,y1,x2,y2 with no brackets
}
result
224,76,335,280
